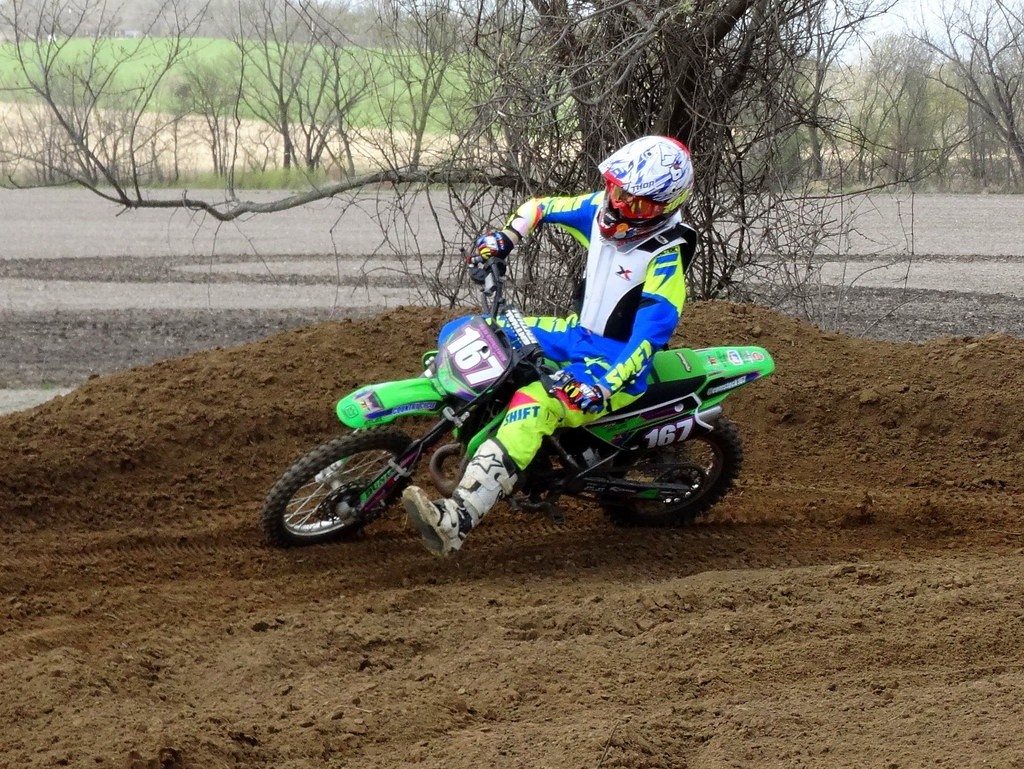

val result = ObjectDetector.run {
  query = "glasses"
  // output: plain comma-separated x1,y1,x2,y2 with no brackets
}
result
605,178,689,220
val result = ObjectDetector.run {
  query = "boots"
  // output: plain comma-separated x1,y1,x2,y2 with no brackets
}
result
401,437,518,560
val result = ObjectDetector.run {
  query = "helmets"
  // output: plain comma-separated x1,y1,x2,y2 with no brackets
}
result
598,136,694,241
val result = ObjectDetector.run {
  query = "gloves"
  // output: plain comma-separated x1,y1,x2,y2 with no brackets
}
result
466,231,513,266
564,383,604,414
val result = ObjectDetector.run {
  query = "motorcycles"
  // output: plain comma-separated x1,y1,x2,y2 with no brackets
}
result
262,236,775,548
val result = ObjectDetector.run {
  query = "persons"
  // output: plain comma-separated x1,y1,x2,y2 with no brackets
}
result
401,136,696,558
